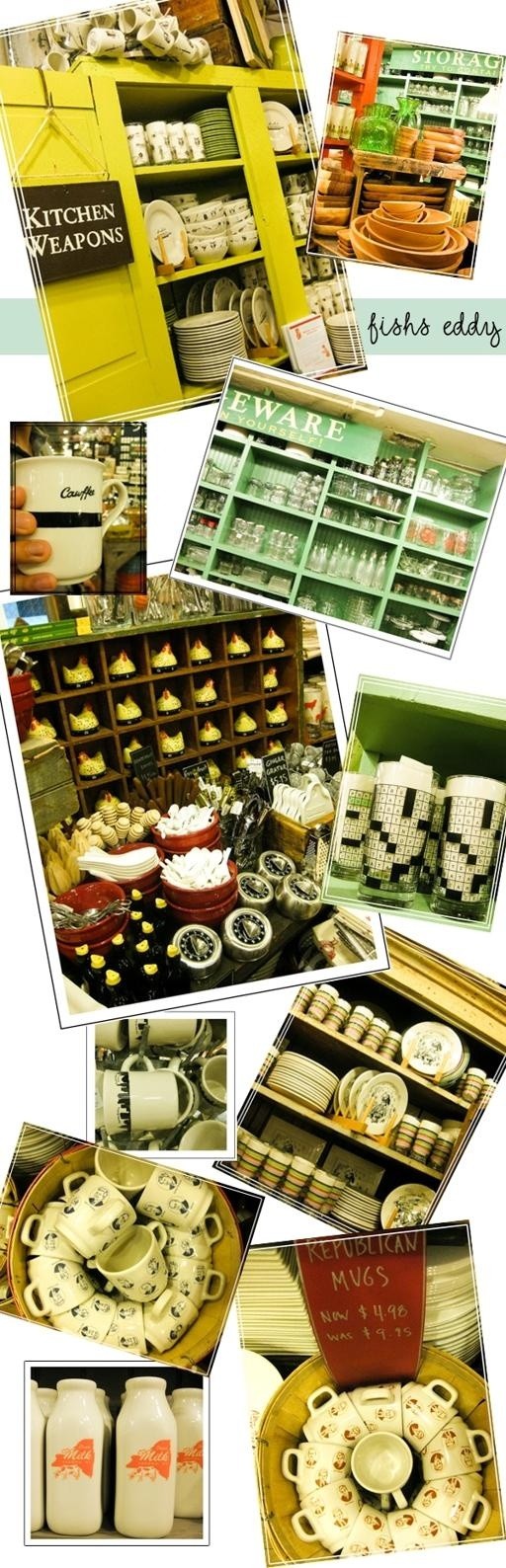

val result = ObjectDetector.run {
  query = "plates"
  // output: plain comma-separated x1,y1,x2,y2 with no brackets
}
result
333,1076,341,1116
12,1124,78,1178
356,1072,409,1136
200,279,215,313
165,308,180,365
362,190,446,203
252,286,279,347
422,130,465,145
172,311,248,383
336,229,352,247
186,108,239,161
348,1069,381,1120
262,100,299,153
363,179,447,194
338,1066,369,1118
239,287,259,350
361,199,444,211
423,1245,480,1368
212,275,238,314
331,1185,382,1233
245,951,283,981
336,245,355,258
228,291,240,313
415,142,435,161
337,240,353,252
398,1020,472,1090
360,208,372,214
423,125,466,138
317,593,374,626
265,1050,341,1114
395,126,420,158
419,139,464,153
144,200,188,267
435,151,462,162
325,310,366,368
141,203,151,215
380,1183,437,1231
186,281,206,317
237,1246,322,1357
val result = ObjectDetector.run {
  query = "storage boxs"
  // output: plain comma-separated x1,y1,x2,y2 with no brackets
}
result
159,2,225,33
265,802,335,857
199,25,246,68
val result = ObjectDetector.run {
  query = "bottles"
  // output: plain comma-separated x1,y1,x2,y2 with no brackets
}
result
103,431,143,529
171,1388,212,1519
310,541,355,578
403,76,493,123
28,1379,46,1533
45,1377,104,1534
398,456,417,489
192,517,218,544
75,888,186,1006
385,456,402,486
242,252,346,321
113,1376,174,1540
355,552,368,587
247,469,325,514
453,125,494,180
362,550,378,586
370,556,386,589
226,515,298,564
375,457,391,482
373,552,389,591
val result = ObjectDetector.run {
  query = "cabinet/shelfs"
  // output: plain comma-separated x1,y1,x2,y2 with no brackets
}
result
58,844,336,1011
327,691,506,929
223,929,506,1233
18,740,81,840
5,610,305,817
182,357,506,659
0,0,362,424
373,47,503,221
108,431,145,510
324,32,387,170
302,621,343,778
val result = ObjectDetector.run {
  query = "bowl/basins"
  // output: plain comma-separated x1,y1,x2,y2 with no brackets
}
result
226,209,254,226
56,913,137,964
177,201,225,224
222,198,249,217
185,216,230,235
8,673,32,695
53,880,126,945
187,237,231,265
315,191,351,200
15,710,33,743
131,884,160,897
317,179,351,196
349,201,468,275
314,206,351,215
161,193,197,205
227,231,258,255
157,830,227,854
231,215,257,234
107,843,165,892
186,229,229,239
313,213,348,225
315,200,348,207
319,168,354,182
313,222,348,237
158,858,239,908
210,194,232,202
149,810,219,852
162,889,238,925
174,202,198,213
11,689,33,714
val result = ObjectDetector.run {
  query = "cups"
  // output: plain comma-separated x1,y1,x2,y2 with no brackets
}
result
319,1174,345,1214
455,1072,468,1098
254,261,269,292
394,1113,420,1153
372,490,393,510
167,121,187,162
126,1019,197,1050
282,170,317,240
97,1135,120,1152
192,1054,228,1109
390,496,407,513
96,1070,105,1130
94,1146,151,1202
409,1119,443,1165
428,774,506,922
90,12,117,28
188,36,211,66
183,122,206,161
302,1385,370,1447
135,1164,215,1234
118,4,155,34
375,1493,389,1508
418,787,444,893
176,580,215,618
157,1019,214,1056
94,1222,169,1302
179,1118,226,1151
362,1018,391,1053
303,1169,334,1210
146,121,172,165
350,1432,408,1509
86,1259,95,1271
87,27,126,58
322,999,351,1032
130,575,174,626
421,1416,495,1481
412,1473,491,1535
339,1505,394,1557
13,456,129,587
163,1255,226,1311
165,28,196,66
387,1509,458,1551
291,1478,361,1555
95,1020,128,1052
124,122,150,167
63,20,90,48
143,1289,199,1353
20,1202,84,1264
23,1256,96,1318
330,472,354,499
378,1030,403,1062
152,1057,200,1128
462,1067,487,1102
373,516,387,534
136,18,175,57
383,520,400,538
344,1005,375,1042
0,1204,19,1249
355,481,376,505
47,1293,117,1344
40,52,70,71
102,1053,177,1133
291,984,318,1015
307,983,339,1022
476,1078,497,1107
231,1135,253,1170
258,1045,279,1085
404,584,461,608
236,1138,268,1179
334,772,375,882
298,1168,319,1201
401,1379,459,1454
282,1156,317,1199
103,1280,114,1290
281,1442,353,1501
240,264,257,288
298,252,354,324
427,1130,455,1171
54,1172,137,1259
102,1301,150,1355
157,1213,224,1264
84,594,132,633
356,761,433,911
347,1383,402,1437
259,1148,287,1189
255,1140,282,1180
330,505,374,530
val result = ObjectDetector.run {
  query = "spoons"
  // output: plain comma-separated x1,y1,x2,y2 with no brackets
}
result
76,847,159,881
158,846,235,888
270,782,307,822
153,803,216,837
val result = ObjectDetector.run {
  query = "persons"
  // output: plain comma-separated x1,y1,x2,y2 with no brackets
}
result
11,422,96,595
